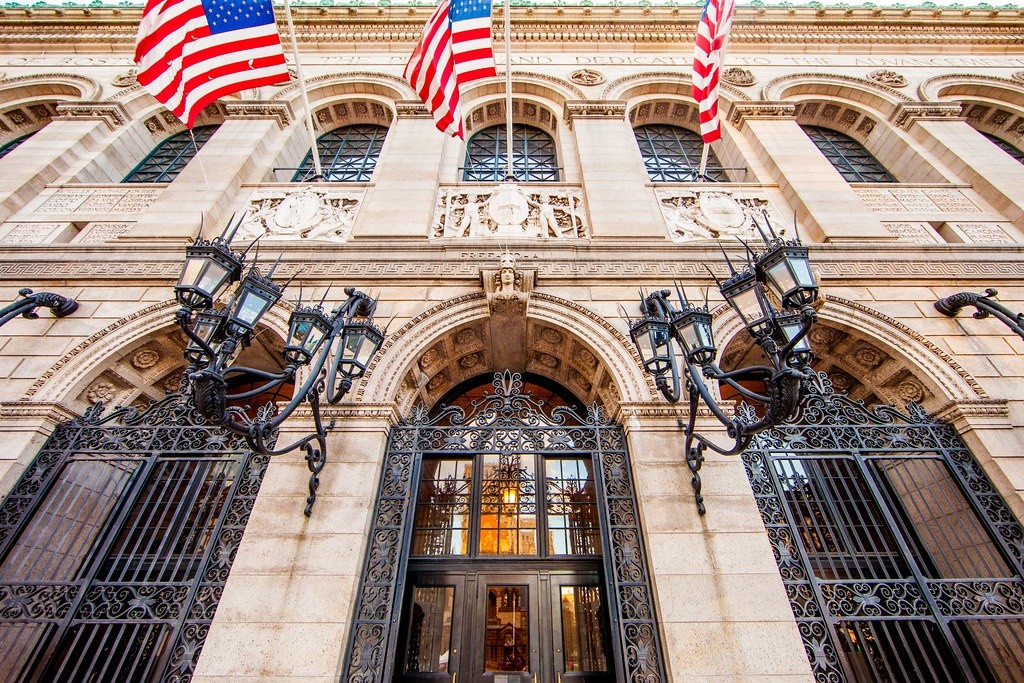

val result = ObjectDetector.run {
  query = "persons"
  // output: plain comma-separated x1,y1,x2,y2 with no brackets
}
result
499,268,516,294
454,193,495,239
517,187,571,238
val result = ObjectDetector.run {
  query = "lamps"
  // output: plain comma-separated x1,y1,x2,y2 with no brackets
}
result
503,485,518,518
616,207,820,518
178,207,402,518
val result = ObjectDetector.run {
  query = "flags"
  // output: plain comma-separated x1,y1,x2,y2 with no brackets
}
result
691,0,735,144
135,0,291,129
403,0,497,141
